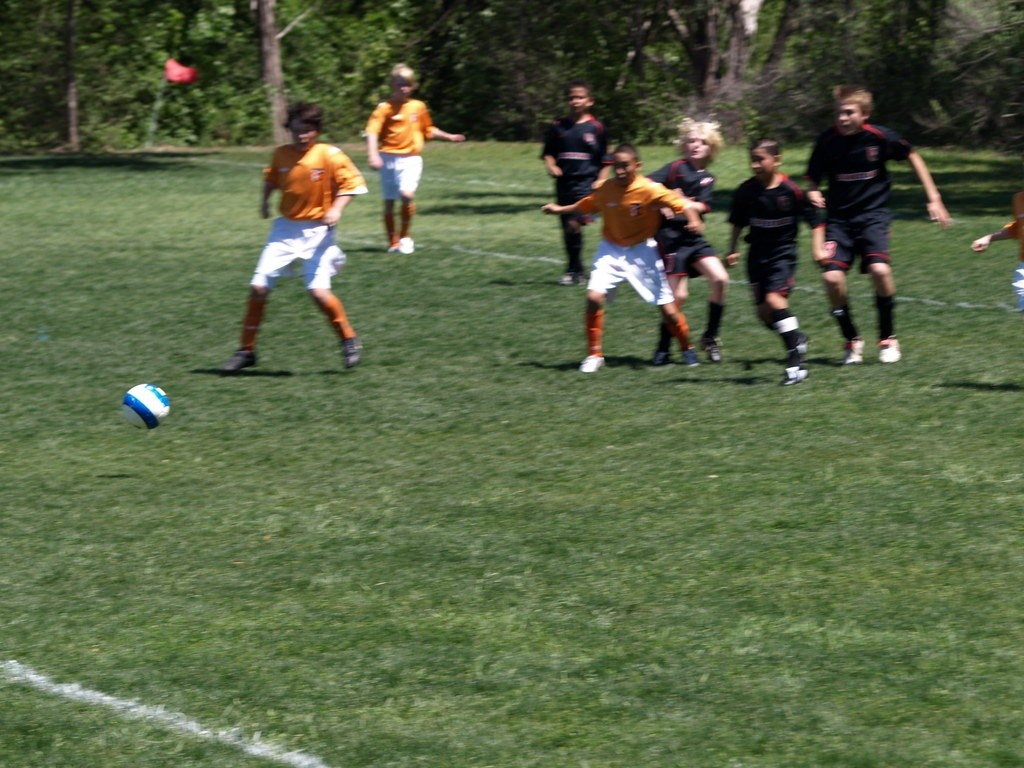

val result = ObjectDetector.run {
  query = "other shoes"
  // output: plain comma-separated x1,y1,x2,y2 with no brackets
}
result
700,338,720,363
560,272,586,285
878,334,901,363
783,363,808,385
684,348,699,366
389,245,400,252
400,236,415,254
844,337,864,365
579,355,604,373
653,350,672,365
785,335,810,376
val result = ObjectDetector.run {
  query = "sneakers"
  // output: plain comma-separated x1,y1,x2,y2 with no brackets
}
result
222,349,257,370
340,336,363,369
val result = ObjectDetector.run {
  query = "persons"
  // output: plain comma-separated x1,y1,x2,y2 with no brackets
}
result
804,83,953,365
969,189,1024,314
641,114,729,366
365,63,465,254
723,135,834,385
539,79,613,285
541,142,705,374
224,98,369,373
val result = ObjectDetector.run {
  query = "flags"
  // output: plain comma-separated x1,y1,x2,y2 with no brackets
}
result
165,56,197,82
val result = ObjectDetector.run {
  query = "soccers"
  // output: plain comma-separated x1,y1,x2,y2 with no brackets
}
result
122,382,171,430
